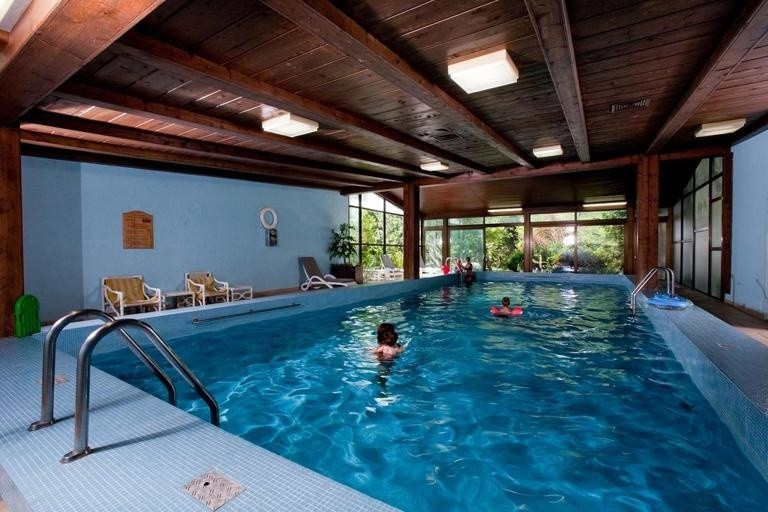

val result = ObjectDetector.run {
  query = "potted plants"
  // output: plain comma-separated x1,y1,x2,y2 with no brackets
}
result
327,221,364,284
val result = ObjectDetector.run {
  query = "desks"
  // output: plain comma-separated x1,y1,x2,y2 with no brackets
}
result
230,286,253,303
162,292,195,308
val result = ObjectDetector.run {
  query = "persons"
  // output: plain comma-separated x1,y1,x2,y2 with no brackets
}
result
500,297,513,312
372,325,399,365
460,257,472,281
454,259,463,273
370,331,404,365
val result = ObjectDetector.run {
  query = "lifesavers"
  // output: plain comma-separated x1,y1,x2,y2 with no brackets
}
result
260,207,278,230
645,291,690,309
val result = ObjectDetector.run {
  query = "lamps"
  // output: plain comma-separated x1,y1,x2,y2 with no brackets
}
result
257,113,319,139
446,48,520,97
532,144,564,159
420,160,449,172
694,116,746,140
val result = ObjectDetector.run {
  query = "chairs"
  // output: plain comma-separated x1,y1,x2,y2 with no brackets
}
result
100,273,162,317
420,256,437,274
298,257,358,292
378,254,402,277
185,273,229,305
